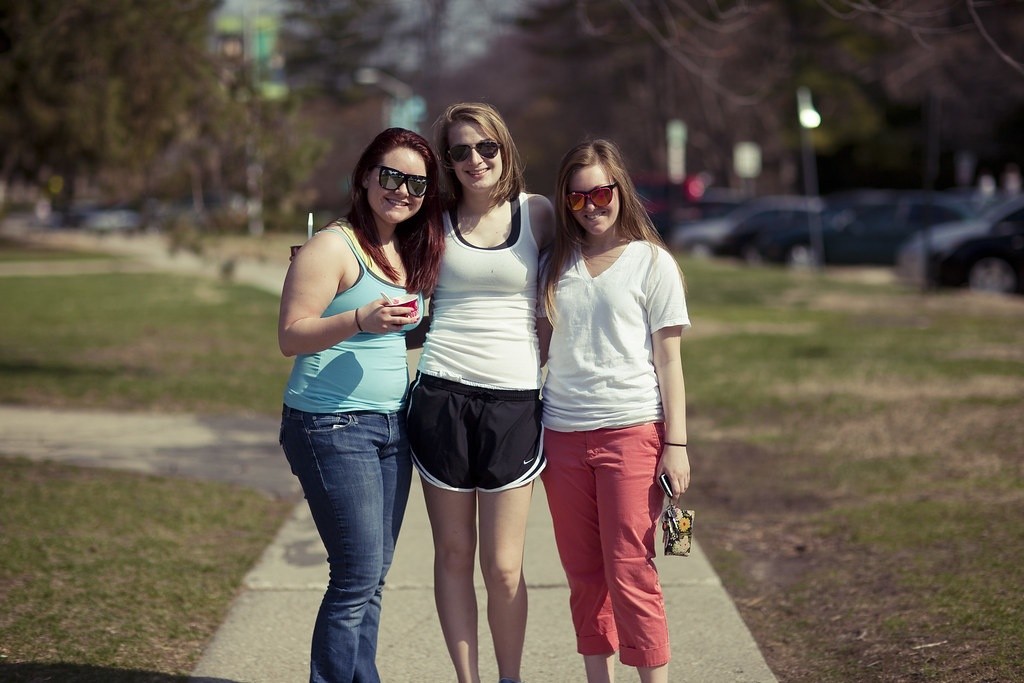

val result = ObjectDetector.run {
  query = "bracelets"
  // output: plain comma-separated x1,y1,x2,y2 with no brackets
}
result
664,442,688,448
356,307,364,334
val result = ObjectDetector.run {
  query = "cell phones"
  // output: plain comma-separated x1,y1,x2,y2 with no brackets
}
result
660,473,673,498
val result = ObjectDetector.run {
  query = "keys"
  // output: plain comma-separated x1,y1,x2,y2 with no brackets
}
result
662,497,681,550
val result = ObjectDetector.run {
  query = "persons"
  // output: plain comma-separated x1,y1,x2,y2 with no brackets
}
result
278,128,446,683
287,103,560,683
428,138,692,683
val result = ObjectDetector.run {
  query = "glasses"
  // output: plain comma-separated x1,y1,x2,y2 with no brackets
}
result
376,165,430,197
566,183,618,212
447,140,501,162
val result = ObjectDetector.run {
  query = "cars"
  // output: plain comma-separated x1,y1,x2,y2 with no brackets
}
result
903,192,1024,292
673,196,824,260
745,190,989,270
634,176,709,224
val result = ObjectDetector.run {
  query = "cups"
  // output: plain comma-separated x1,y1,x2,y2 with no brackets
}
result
385,294,420,325
290,246,303,257
